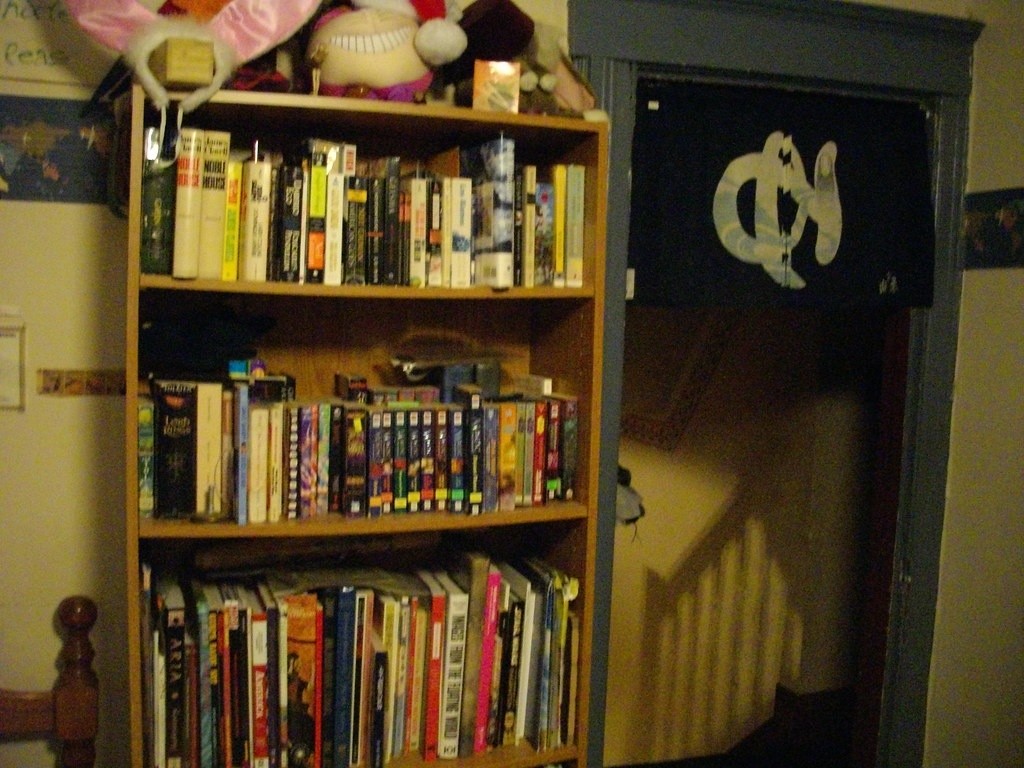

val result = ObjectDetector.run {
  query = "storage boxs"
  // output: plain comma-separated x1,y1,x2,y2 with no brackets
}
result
468,57,525,113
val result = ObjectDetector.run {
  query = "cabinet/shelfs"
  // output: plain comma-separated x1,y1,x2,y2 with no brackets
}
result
102,85,615,768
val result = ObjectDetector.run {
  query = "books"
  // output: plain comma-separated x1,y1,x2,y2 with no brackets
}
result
140,106,590,291
136,350,583,526
137,548,580,768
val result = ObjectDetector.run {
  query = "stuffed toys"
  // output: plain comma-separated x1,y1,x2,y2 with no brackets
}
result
308,0,467,104
